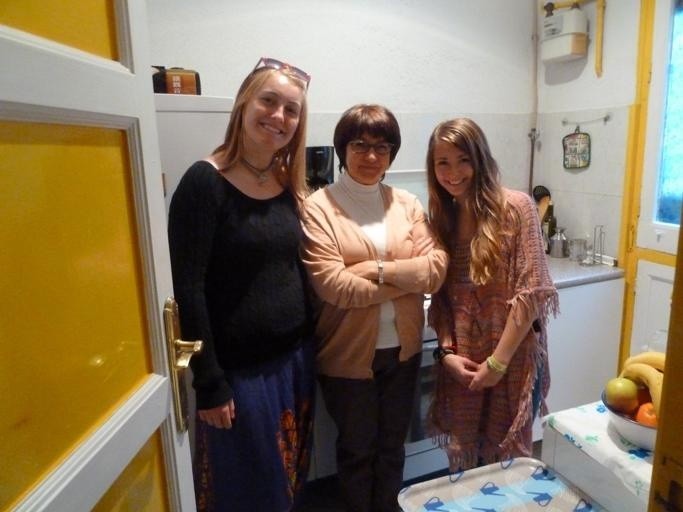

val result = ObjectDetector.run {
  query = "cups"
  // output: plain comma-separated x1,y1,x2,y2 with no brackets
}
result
569,238,588,262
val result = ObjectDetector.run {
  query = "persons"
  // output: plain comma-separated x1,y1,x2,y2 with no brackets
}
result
425,116,559,474
166,60,318,508
299,102,450,512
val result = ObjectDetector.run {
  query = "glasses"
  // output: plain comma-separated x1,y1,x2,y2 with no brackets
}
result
346,138,396,156
252,57,311,95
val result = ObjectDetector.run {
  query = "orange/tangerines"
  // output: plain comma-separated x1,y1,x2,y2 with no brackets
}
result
636,403,658,427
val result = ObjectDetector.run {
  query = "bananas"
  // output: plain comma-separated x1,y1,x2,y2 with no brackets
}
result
619,351,666,418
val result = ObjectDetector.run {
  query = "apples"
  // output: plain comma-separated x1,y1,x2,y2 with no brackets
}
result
605,378,640,412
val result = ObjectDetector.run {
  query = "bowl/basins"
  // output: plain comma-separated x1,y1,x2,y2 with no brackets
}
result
602,377,658,453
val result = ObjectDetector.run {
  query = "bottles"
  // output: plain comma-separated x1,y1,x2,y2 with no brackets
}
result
549,226,567,258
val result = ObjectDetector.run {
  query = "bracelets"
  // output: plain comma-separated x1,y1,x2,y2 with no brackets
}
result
433,345,455,360
485,355,506,373
377,260,385,288
